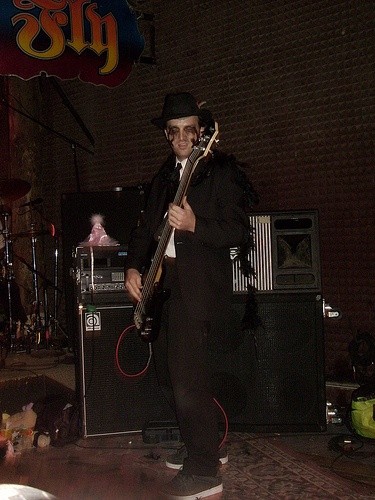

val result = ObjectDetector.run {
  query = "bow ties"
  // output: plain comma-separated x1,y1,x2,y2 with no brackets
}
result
165,162,182,181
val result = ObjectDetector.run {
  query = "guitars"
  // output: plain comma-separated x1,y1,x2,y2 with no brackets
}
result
130,119,220,345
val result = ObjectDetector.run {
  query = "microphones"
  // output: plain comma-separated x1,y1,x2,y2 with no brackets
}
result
18,198,44,208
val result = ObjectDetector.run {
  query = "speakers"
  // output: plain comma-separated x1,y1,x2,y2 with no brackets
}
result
212,291,328,434
76,303,180,438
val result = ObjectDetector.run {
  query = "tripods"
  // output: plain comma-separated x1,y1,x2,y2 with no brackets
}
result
13,206,71,354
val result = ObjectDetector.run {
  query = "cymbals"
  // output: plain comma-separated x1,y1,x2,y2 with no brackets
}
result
0,178,32,205
6,229,52,239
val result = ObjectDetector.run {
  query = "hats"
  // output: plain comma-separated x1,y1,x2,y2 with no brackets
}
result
151,92,212,127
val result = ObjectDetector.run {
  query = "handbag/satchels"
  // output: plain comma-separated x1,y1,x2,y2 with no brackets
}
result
351,394,375,439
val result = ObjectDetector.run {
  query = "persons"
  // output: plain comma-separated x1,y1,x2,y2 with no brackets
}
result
124,92,250,500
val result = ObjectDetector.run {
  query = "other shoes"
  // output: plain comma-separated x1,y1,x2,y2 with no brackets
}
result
165,445,228,469
159,470,224,500
